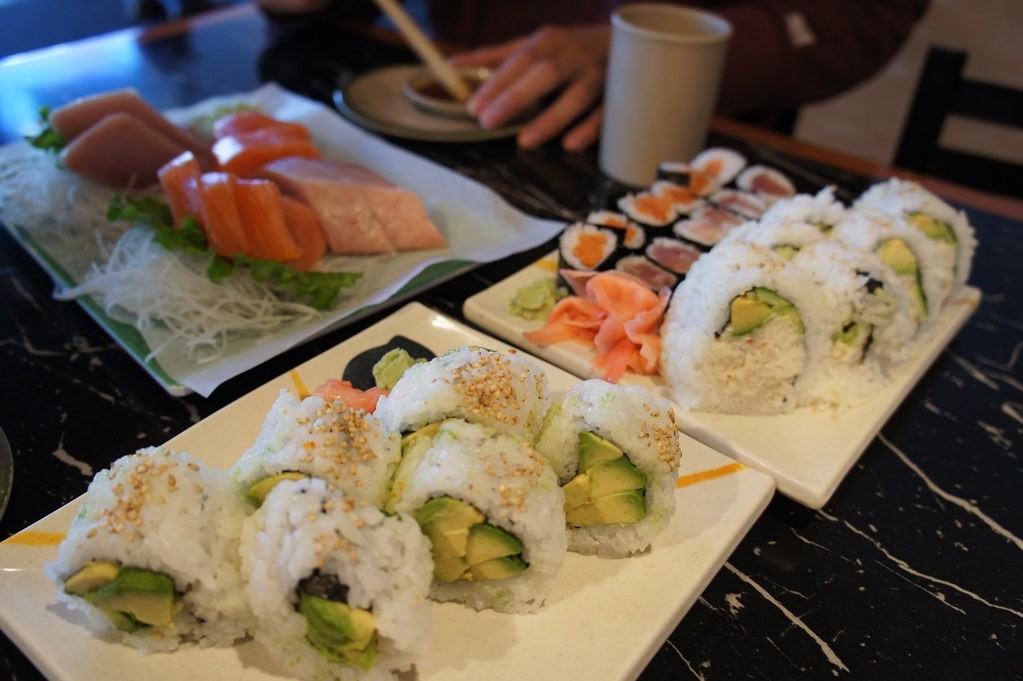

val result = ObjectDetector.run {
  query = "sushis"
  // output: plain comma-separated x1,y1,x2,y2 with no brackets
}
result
46,335,682,681
556,148,976,413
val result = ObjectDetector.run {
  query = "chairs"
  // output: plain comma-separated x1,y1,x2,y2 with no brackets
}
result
890,44,1023,201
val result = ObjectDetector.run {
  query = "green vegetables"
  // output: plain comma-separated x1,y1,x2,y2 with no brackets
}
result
105,190,364,310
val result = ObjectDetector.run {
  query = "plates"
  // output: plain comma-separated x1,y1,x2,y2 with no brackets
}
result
1,302,774,681
0,82,566,396
332,62,545,142
463,248,981,509
402,67,493,117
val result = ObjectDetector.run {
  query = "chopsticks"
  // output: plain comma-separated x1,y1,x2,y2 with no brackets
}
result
378,1,471,103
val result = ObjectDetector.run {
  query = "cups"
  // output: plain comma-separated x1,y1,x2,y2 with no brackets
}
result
600,5,733,187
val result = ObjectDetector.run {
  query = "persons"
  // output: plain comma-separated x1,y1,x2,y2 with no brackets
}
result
261,0,933,151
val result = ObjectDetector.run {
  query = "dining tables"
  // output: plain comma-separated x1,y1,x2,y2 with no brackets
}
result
0,6,1023,680
